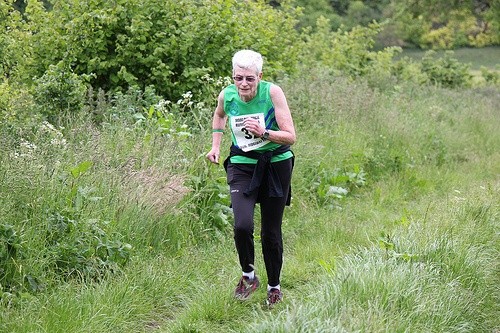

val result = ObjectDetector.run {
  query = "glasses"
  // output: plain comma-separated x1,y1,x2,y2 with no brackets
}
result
232,70,259,82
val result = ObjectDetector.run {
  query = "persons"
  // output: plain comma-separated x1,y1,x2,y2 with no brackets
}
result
202,50,296,306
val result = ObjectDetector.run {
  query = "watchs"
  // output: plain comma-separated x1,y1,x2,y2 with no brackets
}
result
261,130,269,141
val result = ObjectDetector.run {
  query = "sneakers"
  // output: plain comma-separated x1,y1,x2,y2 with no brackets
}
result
266,288,283,310
235,275,260,299
